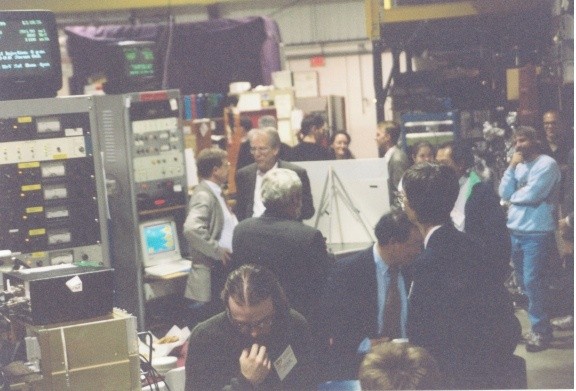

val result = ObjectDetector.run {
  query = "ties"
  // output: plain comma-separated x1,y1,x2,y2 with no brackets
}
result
382,267,403,339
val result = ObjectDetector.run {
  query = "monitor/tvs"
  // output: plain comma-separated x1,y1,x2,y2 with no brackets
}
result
1,8,63,96
138,217,182,269
98,38,161,91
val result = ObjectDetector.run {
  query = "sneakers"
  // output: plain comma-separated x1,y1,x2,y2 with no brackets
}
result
526,332,553,352
551,314,574,330
518,330,536,345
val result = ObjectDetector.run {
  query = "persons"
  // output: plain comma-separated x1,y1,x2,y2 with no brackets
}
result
330,207,424,382
231,167,336,287
329,130,355,159
285,113,336,160
402,161,505,389
412,141,433,163
542,108,573,331
183,148,239,317
236,115,292,169
358,342,441,390
184,264,318,390
500,125,561,353
235,128,315,222
238,116,254,142
434,142,512,280
375,120,409,211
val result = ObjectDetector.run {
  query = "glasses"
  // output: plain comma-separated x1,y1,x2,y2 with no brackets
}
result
246,143,277,157
228,307,275,329
